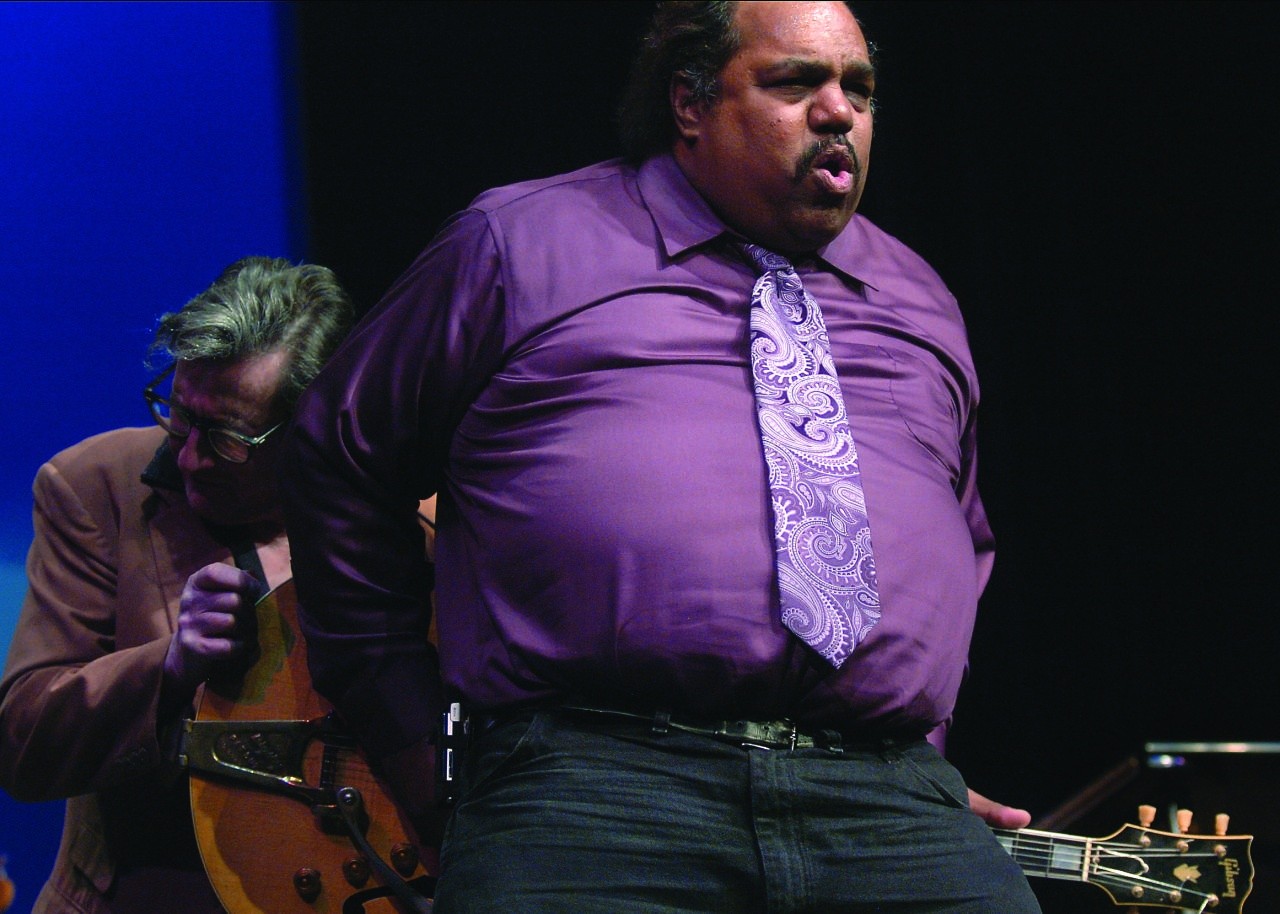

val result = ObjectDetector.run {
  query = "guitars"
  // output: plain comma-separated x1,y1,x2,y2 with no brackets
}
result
188,530,1256,914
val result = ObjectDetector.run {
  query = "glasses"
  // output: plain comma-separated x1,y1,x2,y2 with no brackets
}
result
143,363,285,465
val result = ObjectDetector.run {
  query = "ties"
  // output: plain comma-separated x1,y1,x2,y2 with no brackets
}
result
222,522,269,602
733,237,882,669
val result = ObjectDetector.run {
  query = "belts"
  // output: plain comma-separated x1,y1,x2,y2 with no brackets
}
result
510,684,920,753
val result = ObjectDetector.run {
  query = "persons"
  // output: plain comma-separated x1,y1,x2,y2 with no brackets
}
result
0,255,439,914
280,0,1050,914
